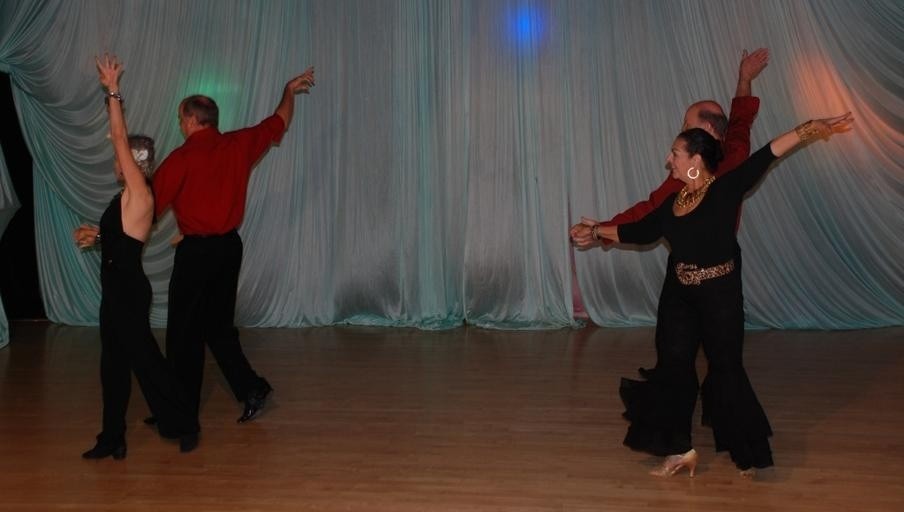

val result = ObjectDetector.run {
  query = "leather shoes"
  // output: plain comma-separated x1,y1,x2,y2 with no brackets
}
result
236,384,274,424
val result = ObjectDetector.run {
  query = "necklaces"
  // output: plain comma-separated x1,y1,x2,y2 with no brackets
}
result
676,175,716,207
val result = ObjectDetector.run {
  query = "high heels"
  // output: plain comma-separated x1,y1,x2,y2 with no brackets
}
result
649,448,698,479
82,440,127,461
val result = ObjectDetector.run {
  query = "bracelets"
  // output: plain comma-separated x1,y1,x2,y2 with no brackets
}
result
794,119,818,142
106,91,123,103
94,231,101,245
592,224,601,241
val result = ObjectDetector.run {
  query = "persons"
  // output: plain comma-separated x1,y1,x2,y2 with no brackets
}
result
568,44,771,378
575,109,855,482
72,63,318,453
79,52,179,460
